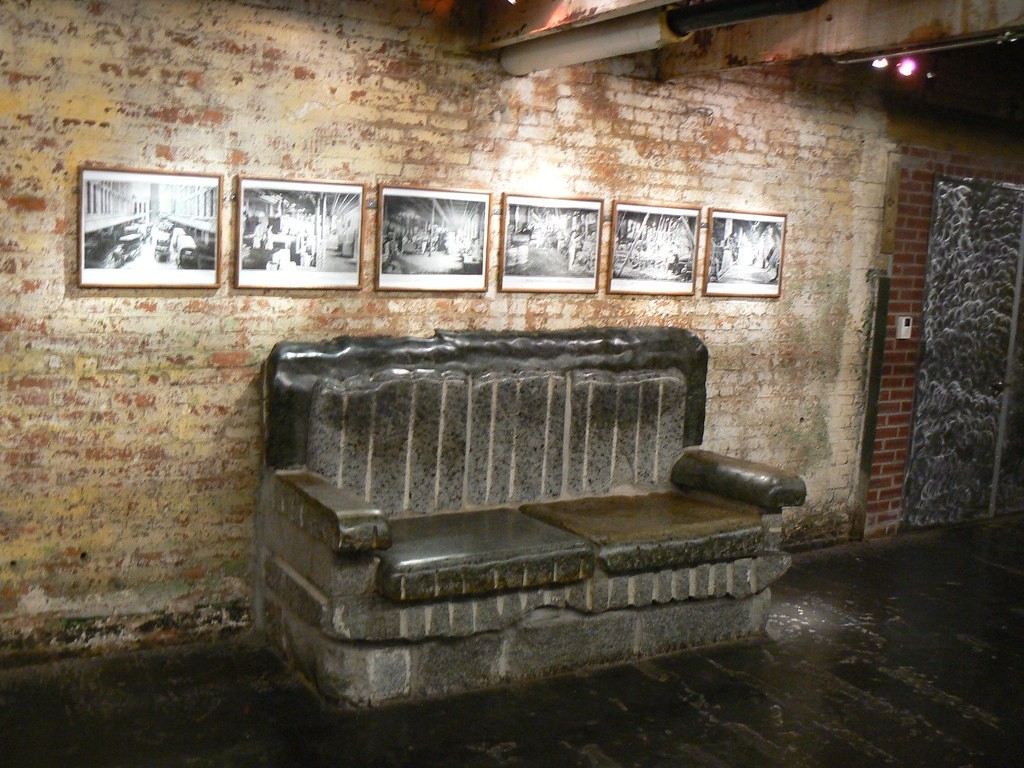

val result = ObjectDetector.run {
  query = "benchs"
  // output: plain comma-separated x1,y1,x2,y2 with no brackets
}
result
253,325,807,710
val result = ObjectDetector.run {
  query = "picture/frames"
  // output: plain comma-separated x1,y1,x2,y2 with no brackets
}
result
234,175,366,292
605,199,702,295
374,182,493,293
76,166,225,290
702,207,789,300
498,191,605,295
